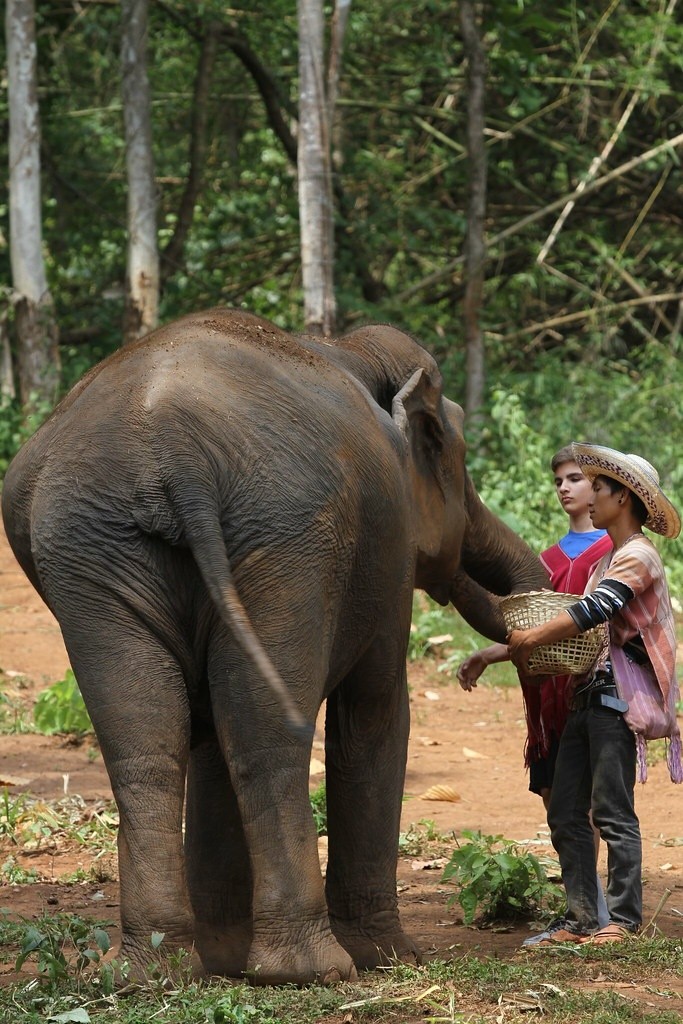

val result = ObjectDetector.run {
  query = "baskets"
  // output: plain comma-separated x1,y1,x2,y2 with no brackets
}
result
496,588,610,676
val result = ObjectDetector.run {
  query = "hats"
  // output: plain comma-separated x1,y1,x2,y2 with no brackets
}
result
570,440,682,539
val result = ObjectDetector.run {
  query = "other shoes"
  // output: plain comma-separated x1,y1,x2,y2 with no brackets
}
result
522,926,560,947
591,924,630,947
539,928,593,948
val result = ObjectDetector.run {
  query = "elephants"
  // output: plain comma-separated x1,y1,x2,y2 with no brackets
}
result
0,307,555,984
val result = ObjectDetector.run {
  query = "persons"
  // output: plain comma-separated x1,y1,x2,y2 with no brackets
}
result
455,442,615,947
506,441,681,951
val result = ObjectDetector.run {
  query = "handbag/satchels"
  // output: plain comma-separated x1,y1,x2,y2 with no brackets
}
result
607,642,683,785
